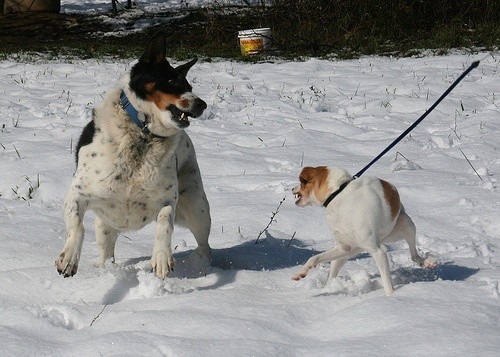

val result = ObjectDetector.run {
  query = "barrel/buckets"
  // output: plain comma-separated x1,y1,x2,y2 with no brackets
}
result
238,28,272,56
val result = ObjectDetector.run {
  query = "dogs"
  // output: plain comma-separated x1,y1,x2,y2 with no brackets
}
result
292,165,437,297
55,37,212,278
